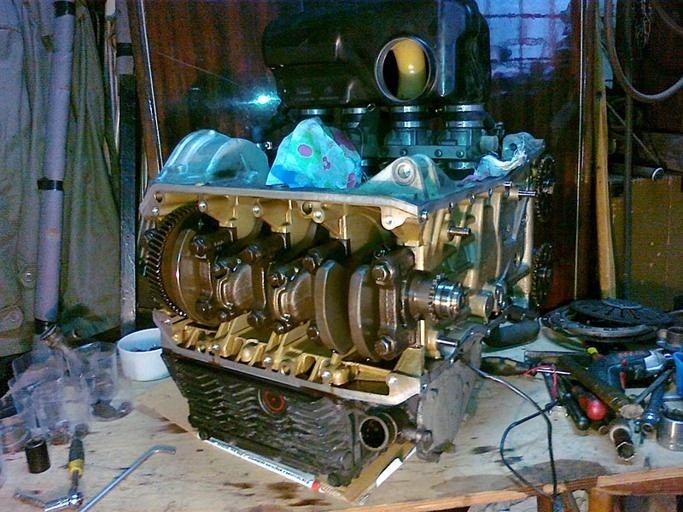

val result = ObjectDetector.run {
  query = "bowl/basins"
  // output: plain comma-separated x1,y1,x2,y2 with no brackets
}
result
116,327,174,383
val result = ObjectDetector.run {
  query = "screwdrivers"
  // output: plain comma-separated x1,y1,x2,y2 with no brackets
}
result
550,367,617,435
69,439,85,501
482,356,572,378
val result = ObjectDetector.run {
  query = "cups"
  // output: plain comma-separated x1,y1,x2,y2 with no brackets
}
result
0,339,120,455
665,327,682,344
656,406,683,453
